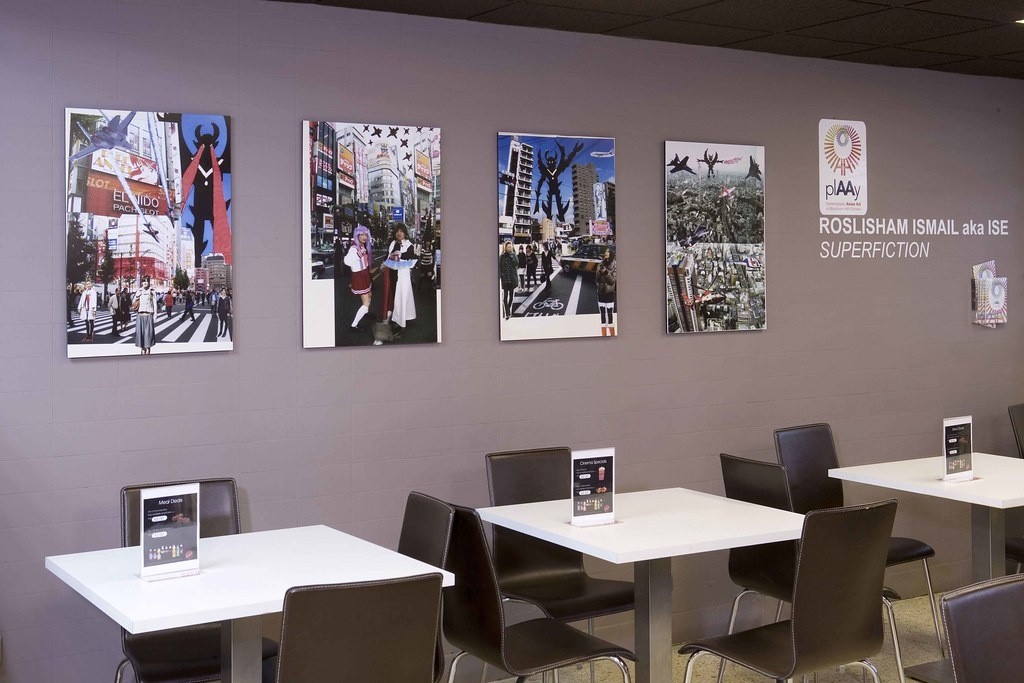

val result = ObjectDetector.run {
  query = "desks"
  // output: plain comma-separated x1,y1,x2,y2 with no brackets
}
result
828,453,1024,683
44,523,455,683
475,488,806,683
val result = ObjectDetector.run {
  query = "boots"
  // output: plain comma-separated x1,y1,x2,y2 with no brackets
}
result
601,323,607,336
607,324,615,337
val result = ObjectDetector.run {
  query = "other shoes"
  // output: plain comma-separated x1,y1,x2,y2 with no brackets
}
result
141,348,150,354
372,340,383,346
351,326,366,332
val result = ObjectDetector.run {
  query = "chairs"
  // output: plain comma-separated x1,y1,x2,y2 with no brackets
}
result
939,573,1024,683
112,477,278,683
717,452,908,683
773,423,945,682
262,490,456,683
1003,402,1024,573
442,504,641,683
676,498,899,683
273,572,444,683
485,446,635,683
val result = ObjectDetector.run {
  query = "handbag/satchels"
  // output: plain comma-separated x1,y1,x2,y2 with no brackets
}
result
80,306,87,321
113,308,121,316
186,302,193,310
211,301,215,305
130,300,139,310
540,271,545,283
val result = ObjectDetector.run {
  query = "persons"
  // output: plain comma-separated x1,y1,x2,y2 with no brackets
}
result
343,224,441,345
500,235,617,337
68,275,232,355
